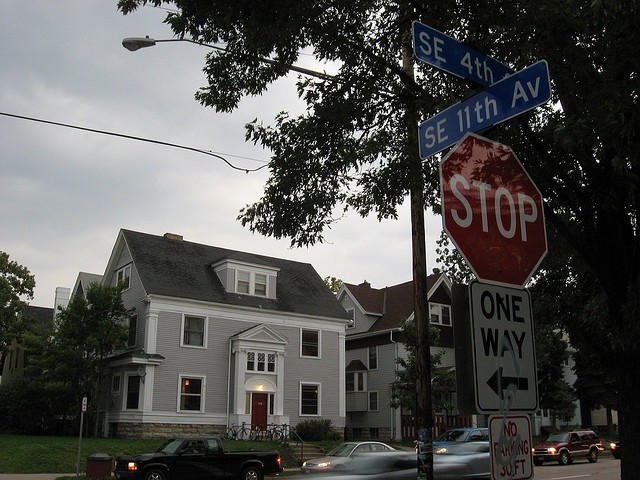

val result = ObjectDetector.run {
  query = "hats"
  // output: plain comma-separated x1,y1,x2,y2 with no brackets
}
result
611,439,621,459
300,441,416,476
429,442,493,478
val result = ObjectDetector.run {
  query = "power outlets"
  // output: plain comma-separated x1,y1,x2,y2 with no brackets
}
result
113,436,286,480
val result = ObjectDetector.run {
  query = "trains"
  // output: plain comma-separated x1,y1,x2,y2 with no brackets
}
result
439,133,550,291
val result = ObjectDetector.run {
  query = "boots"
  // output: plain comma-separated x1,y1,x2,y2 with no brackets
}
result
435,427,489,444
531,429,604,466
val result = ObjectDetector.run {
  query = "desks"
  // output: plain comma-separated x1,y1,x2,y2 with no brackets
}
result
449,283,478,418
463,280,544,415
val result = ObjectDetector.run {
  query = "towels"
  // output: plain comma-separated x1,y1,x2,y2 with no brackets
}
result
86,453,113,476
